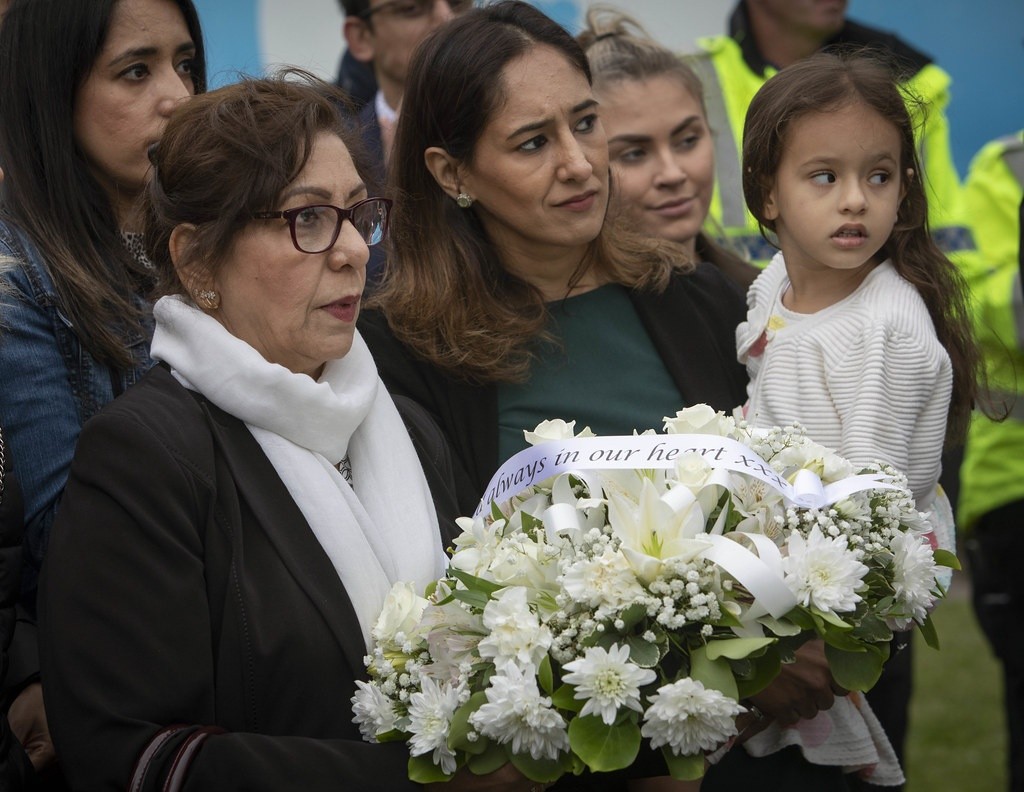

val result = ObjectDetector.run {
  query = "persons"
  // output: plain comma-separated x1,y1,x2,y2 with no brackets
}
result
34,68,546,792
0,0,207,792
332,0,1024,792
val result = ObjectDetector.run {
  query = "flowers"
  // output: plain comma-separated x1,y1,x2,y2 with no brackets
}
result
344,404,953,792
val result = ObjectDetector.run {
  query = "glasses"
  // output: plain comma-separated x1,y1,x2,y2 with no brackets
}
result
359,0,434,20
235,196,393,254
